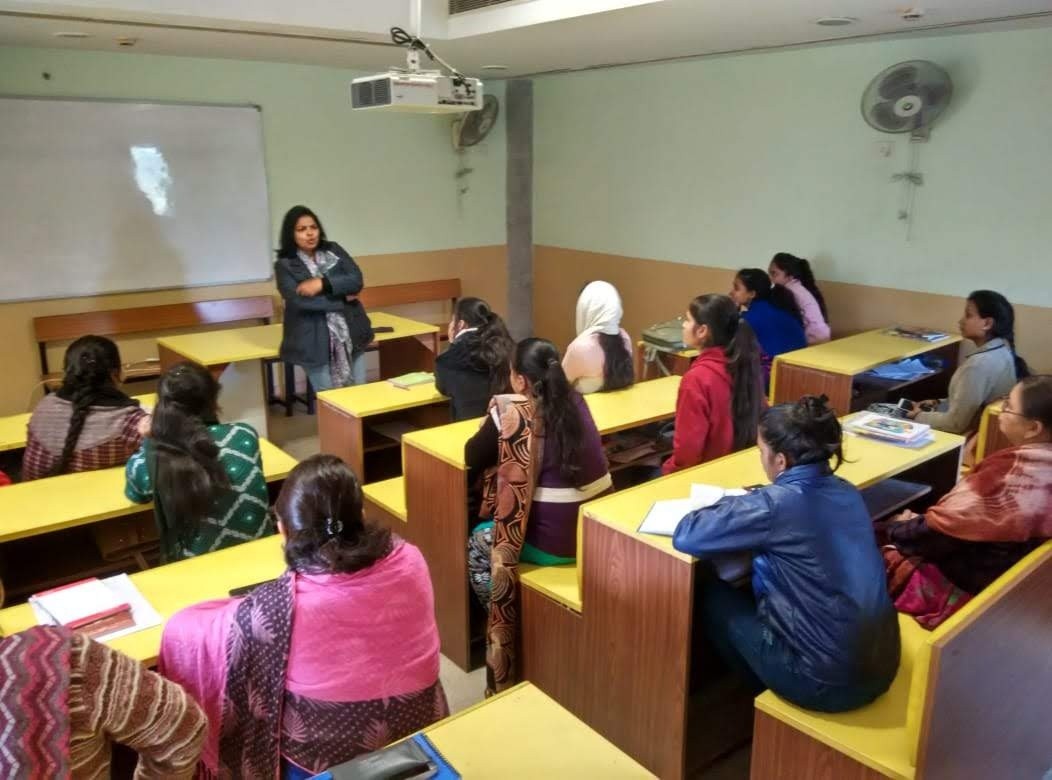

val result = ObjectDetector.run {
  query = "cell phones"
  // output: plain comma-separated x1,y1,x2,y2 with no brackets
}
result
898,398,933,415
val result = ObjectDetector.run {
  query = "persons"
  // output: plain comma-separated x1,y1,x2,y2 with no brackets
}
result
23,334,152,482
124,360,274,563
465,337,614,618
0,582,208,780
731,268,807,396
160,454,450,780
868,290,1030,438
673,394,901,714
433,297,514,424
273,205,374,391
872,375,1052,632
769,253,830,346
628,294,769,487
560,281,634,395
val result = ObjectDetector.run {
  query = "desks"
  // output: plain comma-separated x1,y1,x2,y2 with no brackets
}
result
3,326,1052,780
158,312,441,437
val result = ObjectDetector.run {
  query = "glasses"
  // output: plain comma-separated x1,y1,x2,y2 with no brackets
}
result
1001,400,1038,421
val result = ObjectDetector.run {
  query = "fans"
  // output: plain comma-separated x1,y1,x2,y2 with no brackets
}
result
860,56,953,143
451,92,502,152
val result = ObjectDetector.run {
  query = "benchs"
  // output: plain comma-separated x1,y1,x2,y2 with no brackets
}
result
295,278,466,416
32,296,296,419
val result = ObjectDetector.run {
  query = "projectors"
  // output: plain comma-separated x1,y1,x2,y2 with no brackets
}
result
350,72,485,116
